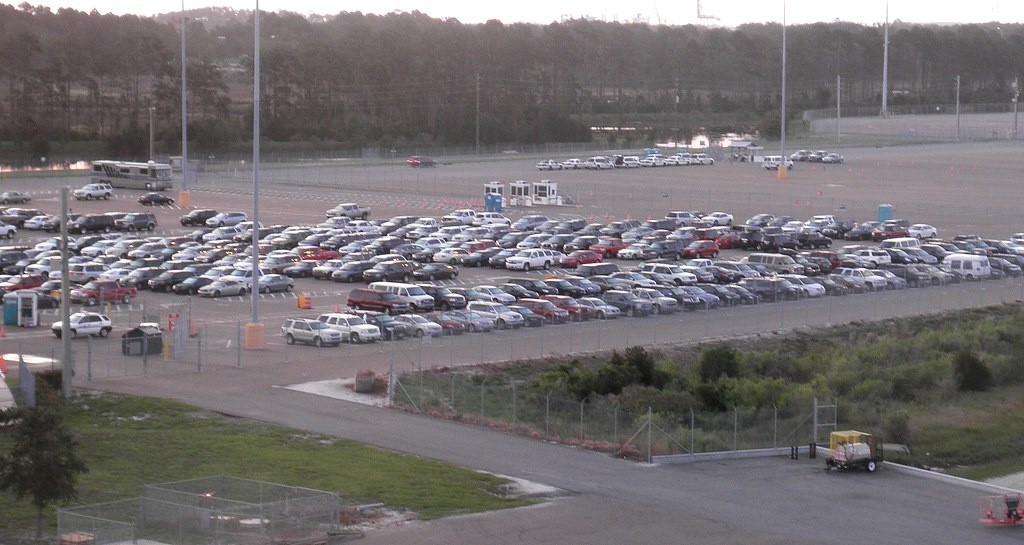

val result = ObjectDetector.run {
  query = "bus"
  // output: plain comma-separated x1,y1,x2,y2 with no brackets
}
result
90,159,173,191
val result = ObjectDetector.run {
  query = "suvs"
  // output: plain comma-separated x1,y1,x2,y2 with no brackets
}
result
346,309,407,341
74,183,113,201
465,300,525,330
518,298,568,325
602,290,653,317
51,310,112,339
540,295,591,321
280,319,341,347
316,312,381,344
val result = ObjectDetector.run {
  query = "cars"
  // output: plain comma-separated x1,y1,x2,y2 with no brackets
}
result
137,192,174,206
508,306,546,327
393,313,442,337
424,313,465,335
0,191,31,204
401,144,845,171
451,309,493,333
0,203,1024,310
576,297,621,320
